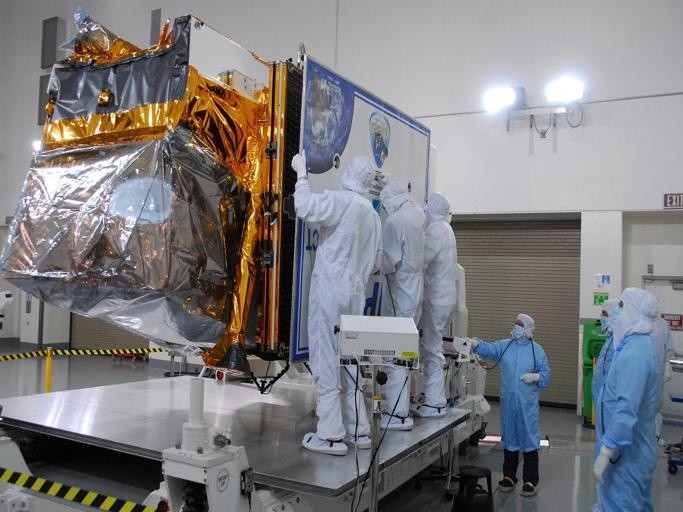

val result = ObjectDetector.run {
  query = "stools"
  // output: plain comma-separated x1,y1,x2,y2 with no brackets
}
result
451,465,493,512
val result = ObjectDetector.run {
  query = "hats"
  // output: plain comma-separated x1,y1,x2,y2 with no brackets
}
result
423,191,449,222
338,156,376,197
379,182,409,216
602,287,659,335
514,314,535,339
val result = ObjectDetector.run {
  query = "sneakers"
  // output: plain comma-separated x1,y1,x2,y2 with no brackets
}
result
302,433,348,456
381,417,413,430
350,435,371,449
414,406,446,418
521,482,539,496
497,478,517,492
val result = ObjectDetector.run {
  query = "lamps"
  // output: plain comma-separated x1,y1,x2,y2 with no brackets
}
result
543,75,589,105
477,84,520,114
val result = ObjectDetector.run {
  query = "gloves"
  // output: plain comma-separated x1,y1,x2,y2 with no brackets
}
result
520,373,540,383
594,446,614,483
290,149,307,180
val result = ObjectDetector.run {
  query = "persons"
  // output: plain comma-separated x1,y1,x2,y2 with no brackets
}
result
409,191,458,418
459,312,551,497
379,178,426,431
589,286,674,512
291,148,384,458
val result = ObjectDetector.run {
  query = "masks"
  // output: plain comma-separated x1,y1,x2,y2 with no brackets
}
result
510,324,524,339
